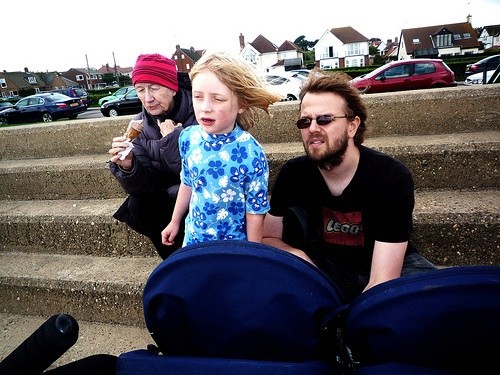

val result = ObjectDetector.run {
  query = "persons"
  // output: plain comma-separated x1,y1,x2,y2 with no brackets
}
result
261,71,438,294
159,48,279,250
109,53,198,261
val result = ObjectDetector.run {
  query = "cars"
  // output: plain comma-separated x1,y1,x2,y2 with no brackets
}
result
263,69,324,102
464,69,500,86
100,88,142,117
98,86,137,107
0,87,91,124
347,58,458,95
464,55,500,78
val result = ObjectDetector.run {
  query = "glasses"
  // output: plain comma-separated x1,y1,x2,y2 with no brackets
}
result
297,114,347,128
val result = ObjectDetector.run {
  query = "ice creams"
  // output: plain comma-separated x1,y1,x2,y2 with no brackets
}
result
126,119,145,142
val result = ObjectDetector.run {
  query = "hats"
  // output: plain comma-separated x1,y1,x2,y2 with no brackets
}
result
132,54,178,91
